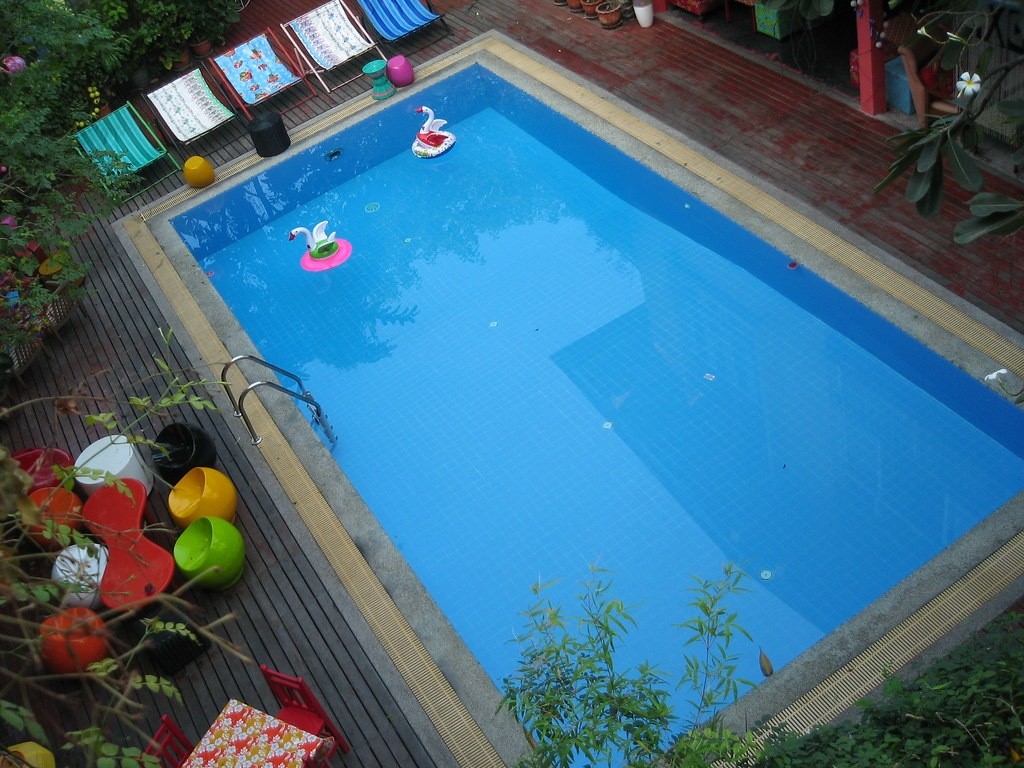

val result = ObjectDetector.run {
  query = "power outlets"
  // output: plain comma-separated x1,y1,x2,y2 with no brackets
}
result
849,44,895,90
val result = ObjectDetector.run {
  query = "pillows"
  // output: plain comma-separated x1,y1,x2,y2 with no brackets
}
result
920,53,954,98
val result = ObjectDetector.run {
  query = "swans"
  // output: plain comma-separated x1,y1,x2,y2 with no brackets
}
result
289,219,339,260
415,105,448,150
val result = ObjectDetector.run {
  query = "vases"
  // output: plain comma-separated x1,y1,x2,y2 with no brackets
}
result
567,0,581,10
553,0,568,6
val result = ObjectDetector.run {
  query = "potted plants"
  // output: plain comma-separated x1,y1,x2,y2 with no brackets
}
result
580,0,602,16
633,0,654,28
58,0,213,116
621,0,634,19
595,0,623,25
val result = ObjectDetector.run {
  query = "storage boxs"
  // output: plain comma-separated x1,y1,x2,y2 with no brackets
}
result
884,56,915,116
754,0,799,41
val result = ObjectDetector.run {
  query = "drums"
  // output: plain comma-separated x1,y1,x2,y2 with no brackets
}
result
362,59,397,100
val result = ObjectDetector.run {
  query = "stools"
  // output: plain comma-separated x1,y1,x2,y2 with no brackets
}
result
387,55,415,88
51,542,108,608
167,467,239,525
362,59,397,100
182,156,215,188
173,516,246,591
21,487,83,545
248,111,291,158
73,435,154,496
40,607,109,673
10,447,73,496
151,423,217,485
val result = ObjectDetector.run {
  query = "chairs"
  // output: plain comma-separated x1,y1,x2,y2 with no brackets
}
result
896,18,965,145
67,101,183,206
279,0,388,93
352,0,455,58
259,663,350,752
134,714,195,768
207,27,318,121
725,0,757,31
139,61,247,161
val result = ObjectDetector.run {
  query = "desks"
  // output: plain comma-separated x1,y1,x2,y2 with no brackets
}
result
81,477,175,611
181,698,335,768
